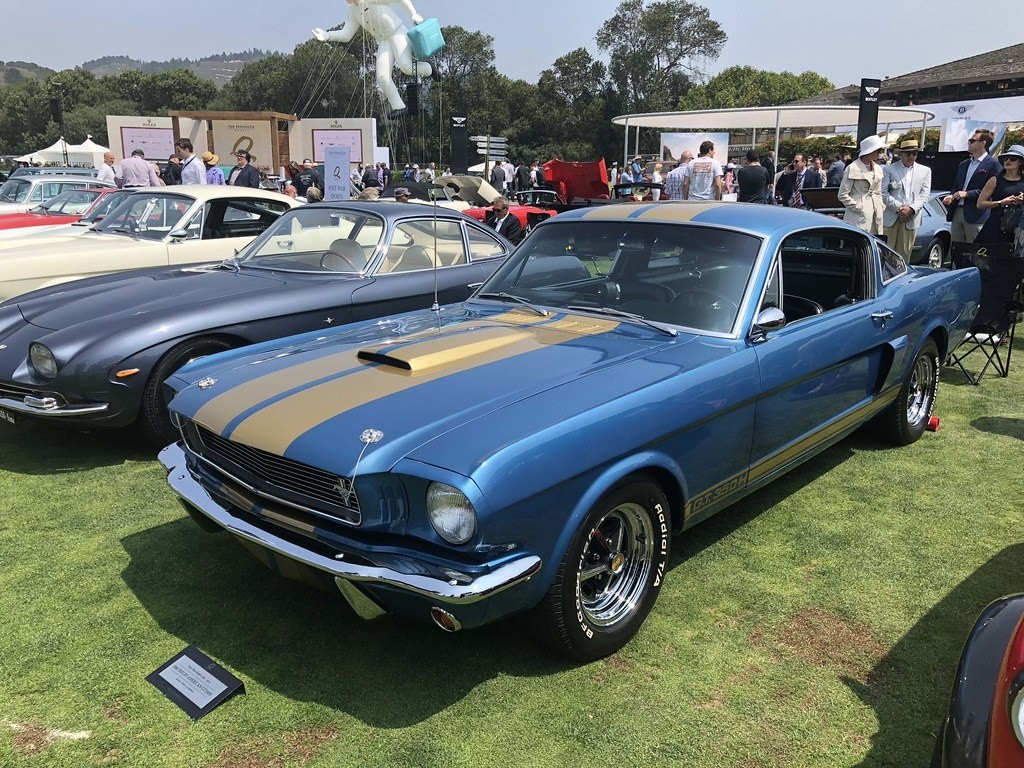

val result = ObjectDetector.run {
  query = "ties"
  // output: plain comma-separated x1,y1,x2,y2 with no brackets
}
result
493,219,500,231
795,174,802,208
111,166,117,174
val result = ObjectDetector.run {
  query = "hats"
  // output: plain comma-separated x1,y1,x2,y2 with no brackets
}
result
857,135,886,157
202,151,220,165
635,155,641,160
303,158,311,163
894,140,920,151
169,154,175,160
394,187,412,197
998,144,1024,165
534,157,540,161
404,163,410,168
412,164,419,169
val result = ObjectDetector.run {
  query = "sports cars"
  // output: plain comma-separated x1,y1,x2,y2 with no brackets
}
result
0,199,594,452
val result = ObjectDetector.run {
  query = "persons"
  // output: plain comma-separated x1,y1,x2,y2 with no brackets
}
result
94,128,1024,344
311,0,444,119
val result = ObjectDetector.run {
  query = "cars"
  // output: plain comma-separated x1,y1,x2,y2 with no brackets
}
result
1,171,671,311
909,188,956,270
154,198,984,659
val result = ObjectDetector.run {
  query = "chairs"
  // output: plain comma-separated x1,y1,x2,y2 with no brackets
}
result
156,207,183,227
945,241,1024,385
328,238,367,271
389,246,442,272
762,294,822,321
619,279,676,318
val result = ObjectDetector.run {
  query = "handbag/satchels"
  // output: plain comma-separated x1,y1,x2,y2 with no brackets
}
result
1000,204,1024,241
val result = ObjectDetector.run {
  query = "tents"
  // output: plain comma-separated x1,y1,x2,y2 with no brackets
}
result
13,132,110,171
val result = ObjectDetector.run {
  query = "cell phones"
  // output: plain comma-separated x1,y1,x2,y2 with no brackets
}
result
789,164,794,170
1019,193,1024,198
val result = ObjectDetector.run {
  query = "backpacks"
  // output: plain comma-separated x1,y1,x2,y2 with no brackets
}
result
534,170,544,185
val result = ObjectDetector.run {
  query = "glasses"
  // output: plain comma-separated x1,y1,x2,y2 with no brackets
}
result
968,139,982,144
904,150,917,156
793,160,802,163
1002,155,1020,161
692,157,694,159
404,195,408,197
360,167,363,169
492,206,506,213
238,155,247,159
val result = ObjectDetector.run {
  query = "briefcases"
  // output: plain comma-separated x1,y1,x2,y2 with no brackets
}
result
407,17,446,60
